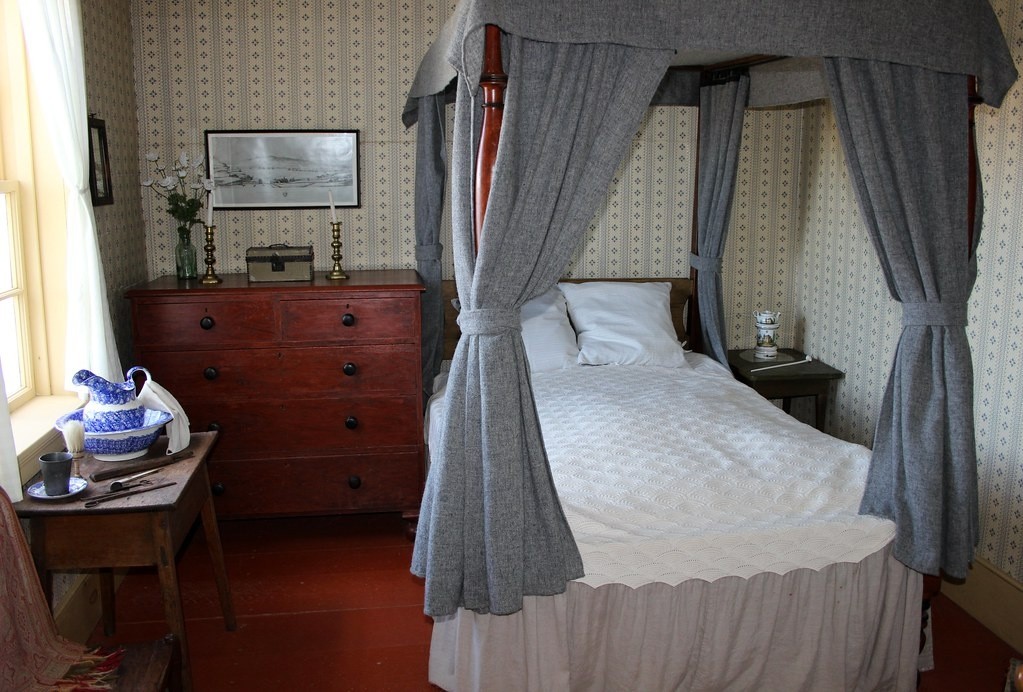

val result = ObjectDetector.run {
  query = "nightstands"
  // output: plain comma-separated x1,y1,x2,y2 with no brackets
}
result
727,348,842,433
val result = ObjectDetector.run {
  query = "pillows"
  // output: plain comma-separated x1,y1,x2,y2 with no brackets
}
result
519,286,582,374
557,282,690,370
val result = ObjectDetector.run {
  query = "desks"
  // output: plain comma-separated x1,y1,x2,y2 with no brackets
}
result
14,431,237,692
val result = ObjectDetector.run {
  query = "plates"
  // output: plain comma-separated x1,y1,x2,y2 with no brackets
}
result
28,478,88,500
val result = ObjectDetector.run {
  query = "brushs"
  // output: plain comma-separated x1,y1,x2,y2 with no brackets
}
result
62,420,86,481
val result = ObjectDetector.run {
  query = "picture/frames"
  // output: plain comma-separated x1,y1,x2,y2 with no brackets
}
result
87,116,114,208
204,129,361,211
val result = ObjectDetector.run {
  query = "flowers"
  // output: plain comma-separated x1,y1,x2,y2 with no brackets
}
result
141,152,214,278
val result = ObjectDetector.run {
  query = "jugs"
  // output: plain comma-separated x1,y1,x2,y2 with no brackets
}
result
72,366,151,432
753,310,782,324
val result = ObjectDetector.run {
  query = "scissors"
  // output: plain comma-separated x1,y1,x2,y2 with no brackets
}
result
103,479,152,494
79,482,177,508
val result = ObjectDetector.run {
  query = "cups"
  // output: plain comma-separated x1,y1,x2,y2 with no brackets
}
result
39,452,73,495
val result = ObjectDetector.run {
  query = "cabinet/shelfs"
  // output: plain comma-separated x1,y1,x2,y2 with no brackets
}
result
122,269,427,540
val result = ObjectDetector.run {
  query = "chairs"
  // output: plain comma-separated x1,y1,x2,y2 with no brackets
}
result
0,485,184,692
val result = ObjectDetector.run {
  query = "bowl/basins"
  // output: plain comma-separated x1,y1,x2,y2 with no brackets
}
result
55,408,174,461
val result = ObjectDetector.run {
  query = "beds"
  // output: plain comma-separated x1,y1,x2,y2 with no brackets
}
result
403,1,934,692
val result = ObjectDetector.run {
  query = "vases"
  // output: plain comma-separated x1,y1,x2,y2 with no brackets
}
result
175,233,198,279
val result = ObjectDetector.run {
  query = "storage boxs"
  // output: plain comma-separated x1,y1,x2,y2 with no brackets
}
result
245,244,315,283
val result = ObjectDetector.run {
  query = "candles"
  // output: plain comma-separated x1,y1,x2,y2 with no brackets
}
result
328,189,338,224
207,193,213,228
